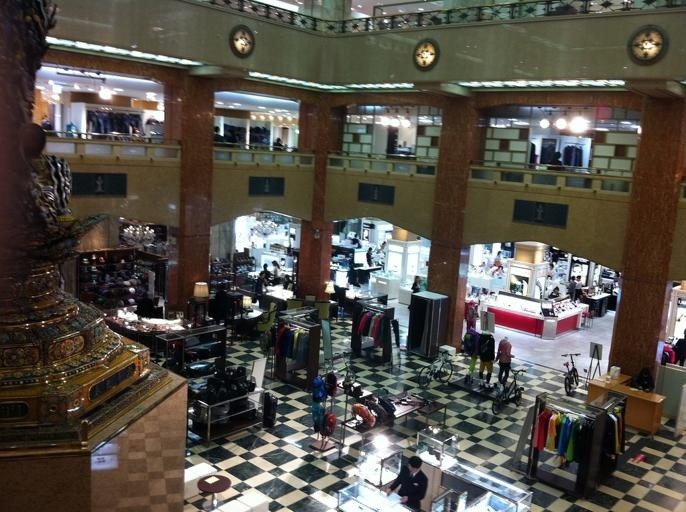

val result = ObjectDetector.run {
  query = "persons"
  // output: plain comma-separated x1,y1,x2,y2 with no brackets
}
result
635,368,655,392
40,120,58,137
213,127,227,142
342,227,386,288
462,300,516,387
672,328,686,366
492,251,504,276
386,455,428,512
255,261,294,293
548,152,562,171
549,275,583,300
274,138,286,151
408,276,421,309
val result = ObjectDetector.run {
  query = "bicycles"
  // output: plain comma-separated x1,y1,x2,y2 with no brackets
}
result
492,367,527,414
418,350,454,388
561,353,582,396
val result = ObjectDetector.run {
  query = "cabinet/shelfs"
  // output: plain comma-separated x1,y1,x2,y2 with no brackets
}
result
262,319,322,385
356,253,623,338
335,439,534,512
74,246,355,320
105,320,258,446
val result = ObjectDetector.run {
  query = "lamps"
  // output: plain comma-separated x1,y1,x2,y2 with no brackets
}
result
121,224,156,247
538,106,596,136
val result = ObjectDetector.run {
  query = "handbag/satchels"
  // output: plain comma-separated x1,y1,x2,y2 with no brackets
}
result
313,403,337,436
352,395,396,427
312,372,338,402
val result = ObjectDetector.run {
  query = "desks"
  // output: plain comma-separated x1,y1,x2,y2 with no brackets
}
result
416,425,460,456
197,474,231,512
340,393,426,452
397,391,448,427
